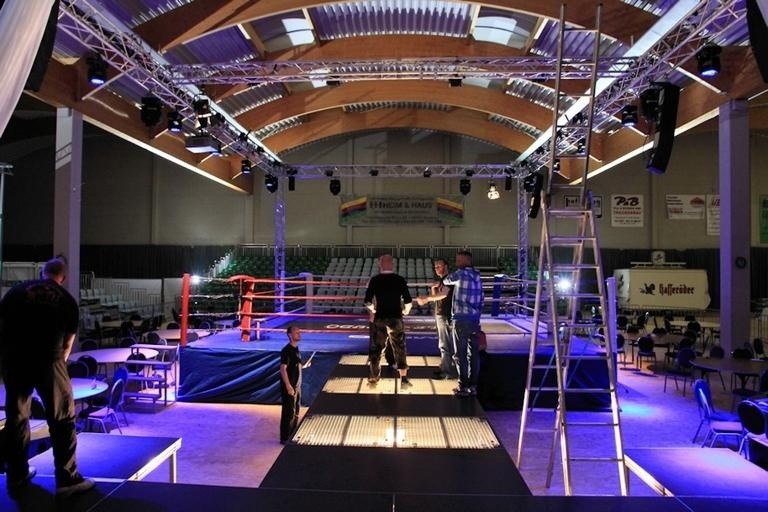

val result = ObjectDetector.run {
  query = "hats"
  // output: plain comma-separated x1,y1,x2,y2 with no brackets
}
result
380,253,393,272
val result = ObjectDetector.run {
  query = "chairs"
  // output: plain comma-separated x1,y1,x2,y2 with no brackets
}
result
568,299,768,473
30,287,237,434
190,254,441,314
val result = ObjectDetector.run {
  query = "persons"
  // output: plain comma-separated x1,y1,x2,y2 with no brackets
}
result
417,258,458,379
0,259,95,494
364,254,413,389
280,327,302,444
443,252,484,396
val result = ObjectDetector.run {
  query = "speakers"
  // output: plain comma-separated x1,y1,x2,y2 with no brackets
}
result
643,83,681,176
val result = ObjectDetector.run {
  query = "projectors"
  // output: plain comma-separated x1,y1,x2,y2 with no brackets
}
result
185,135,220,155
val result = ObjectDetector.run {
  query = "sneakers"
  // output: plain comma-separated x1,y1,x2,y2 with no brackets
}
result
7,466,36,491
400,381,413,387
452,384,477,396
54,476,94,494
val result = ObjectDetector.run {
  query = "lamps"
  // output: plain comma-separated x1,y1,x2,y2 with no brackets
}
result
425,167,433,178
140,95,227,154
488,173,500,200
620,104,640,126
460,171,477,194
553,158,563,172
504,167,517,192
241,158,251,173
330,178,341,195
88,57,108,84
694,43,723,77
578,138,588,152
287,169,297,190
266,174,280,192
450,72,464,87
327,73,341,86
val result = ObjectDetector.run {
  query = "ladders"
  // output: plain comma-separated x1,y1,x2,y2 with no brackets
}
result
516,0,629,496
